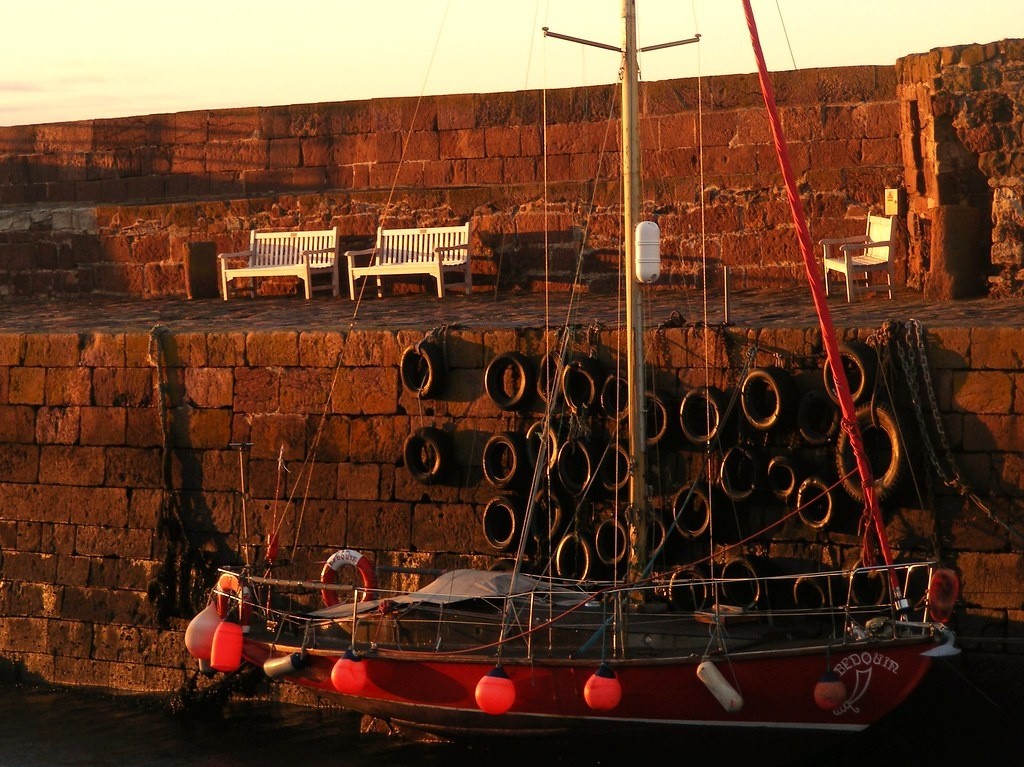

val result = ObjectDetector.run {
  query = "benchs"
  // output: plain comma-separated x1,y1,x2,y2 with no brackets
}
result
345,221,470,300
217,226,339,301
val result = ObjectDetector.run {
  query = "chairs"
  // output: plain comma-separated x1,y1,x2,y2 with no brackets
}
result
818,210,895,303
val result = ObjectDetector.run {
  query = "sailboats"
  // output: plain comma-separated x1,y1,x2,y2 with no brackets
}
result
182,0,966,751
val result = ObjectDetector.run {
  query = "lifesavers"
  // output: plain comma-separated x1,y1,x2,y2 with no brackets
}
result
928,568,960,624
402,426,454,485
318,549,375,609
217,574,251,636
399,343,443,399
482,344,907,615
